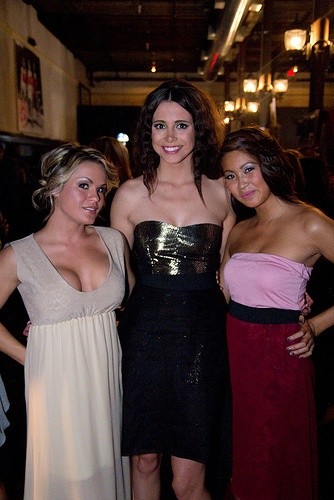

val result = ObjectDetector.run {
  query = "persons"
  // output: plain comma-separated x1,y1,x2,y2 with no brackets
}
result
23,82,313,500
0,143,158,500
219,129,333,500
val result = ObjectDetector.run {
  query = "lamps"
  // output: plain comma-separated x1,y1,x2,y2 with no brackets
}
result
218,10,329,127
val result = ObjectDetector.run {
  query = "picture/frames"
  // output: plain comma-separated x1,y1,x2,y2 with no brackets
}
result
78,82,91,105
12,37,49,137
